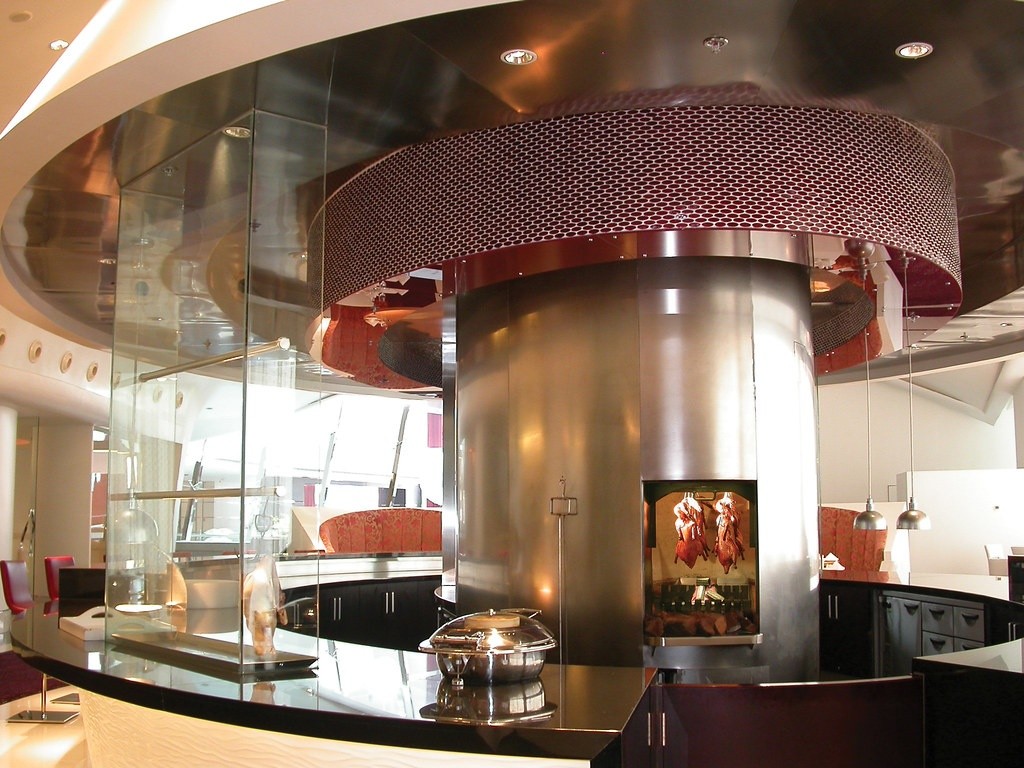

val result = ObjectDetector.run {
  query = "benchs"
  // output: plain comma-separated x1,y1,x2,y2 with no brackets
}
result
319,507,441,551
818,506,889,582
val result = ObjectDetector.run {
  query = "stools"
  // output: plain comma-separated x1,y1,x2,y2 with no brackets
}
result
1,560,80,724
44,555,80,704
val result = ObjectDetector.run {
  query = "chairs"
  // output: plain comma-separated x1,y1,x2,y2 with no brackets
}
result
985,544,1008,575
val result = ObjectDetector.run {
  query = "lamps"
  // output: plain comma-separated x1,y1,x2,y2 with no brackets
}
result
851,239,888,532
894,255,931,529
